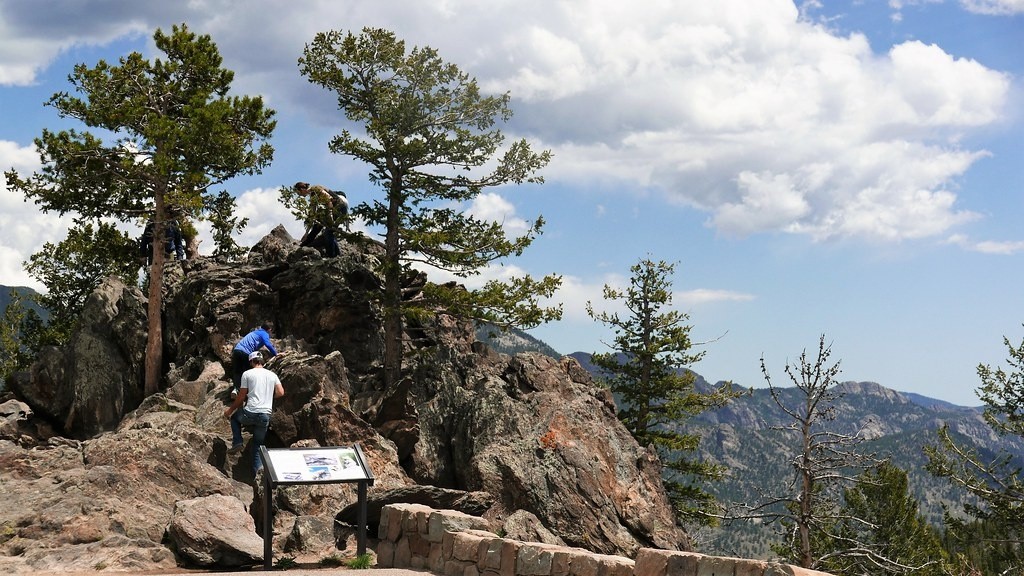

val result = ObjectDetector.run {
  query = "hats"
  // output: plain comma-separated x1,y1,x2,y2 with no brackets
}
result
249,351,263,361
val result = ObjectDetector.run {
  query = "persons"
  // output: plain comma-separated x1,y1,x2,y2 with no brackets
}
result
225,351,285,485
231,322,284,400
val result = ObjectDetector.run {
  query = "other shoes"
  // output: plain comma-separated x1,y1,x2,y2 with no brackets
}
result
231,389,237,400
227,442,244,455
